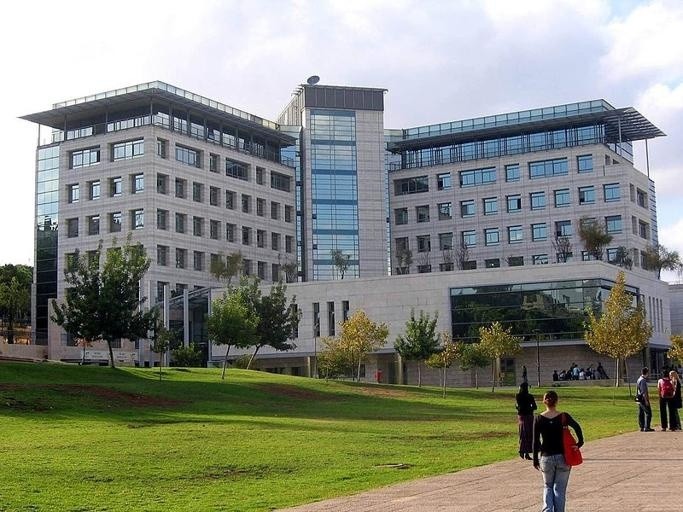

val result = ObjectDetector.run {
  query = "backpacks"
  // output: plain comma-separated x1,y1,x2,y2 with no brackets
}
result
661,378,674,396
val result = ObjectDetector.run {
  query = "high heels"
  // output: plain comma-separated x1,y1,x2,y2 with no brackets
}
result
519,451,524,459
525,454,532,460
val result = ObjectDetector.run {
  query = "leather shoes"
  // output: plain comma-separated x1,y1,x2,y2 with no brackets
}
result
644,428,655,432
661,426,681,431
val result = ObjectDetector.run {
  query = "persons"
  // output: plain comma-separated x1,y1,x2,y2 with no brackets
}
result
552,362,609,380
522,365,527,382
375,368,382,383
515,382,537,459
668,371,683,430
656,369,675,431
635,367,654,431
532,391,584,511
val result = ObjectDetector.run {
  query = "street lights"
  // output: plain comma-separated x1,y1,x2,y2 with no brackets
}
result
531,328,540,387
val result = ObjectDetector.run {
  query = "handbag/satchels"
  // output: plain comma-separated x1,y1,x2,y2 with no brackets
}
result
561,413,583,466
634,395,644,403
